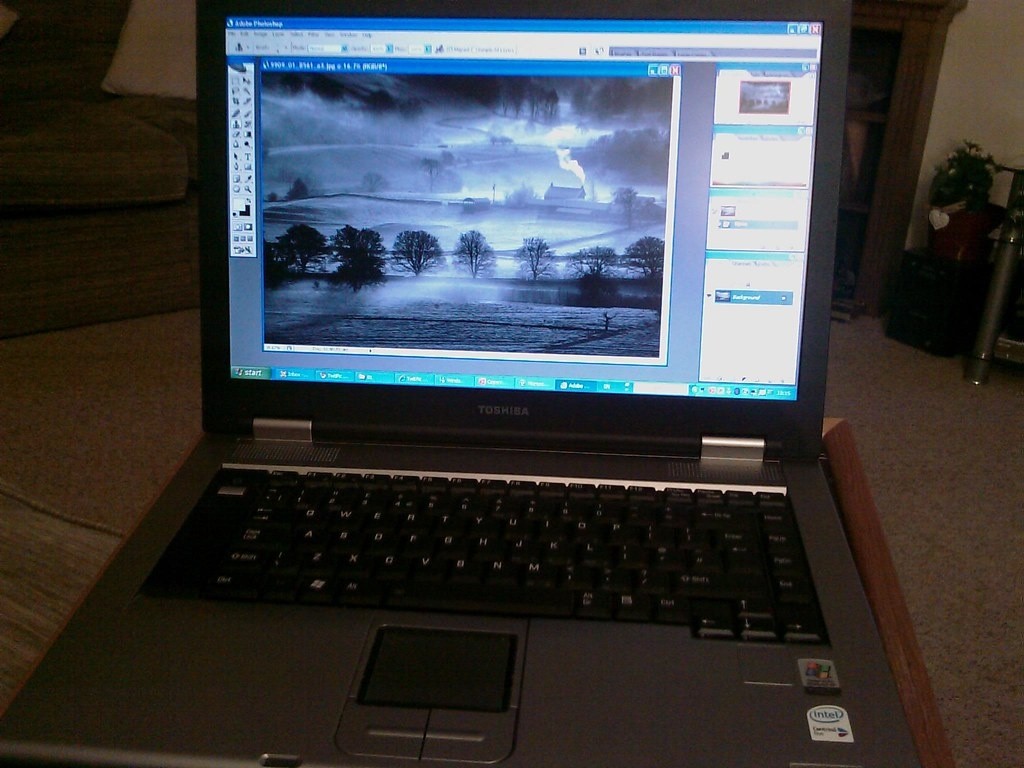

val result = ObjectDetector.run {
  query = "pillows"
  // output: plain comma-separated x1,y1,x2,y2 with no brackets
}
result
97,0,198,101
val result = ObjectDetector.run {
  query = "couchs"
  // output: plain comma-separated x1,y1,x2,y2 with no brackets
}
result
0,0,201,336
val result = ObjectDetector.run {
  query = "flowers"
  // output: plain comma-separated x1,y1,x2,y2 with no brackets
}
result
928,137,1003,214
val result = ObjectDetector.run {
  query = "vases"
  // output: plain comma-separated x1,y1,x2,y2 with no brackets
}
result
926,203,1004,261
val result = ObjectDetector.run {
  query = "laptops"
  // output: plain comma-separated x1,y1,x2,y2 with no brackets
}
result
0,0,918,768
